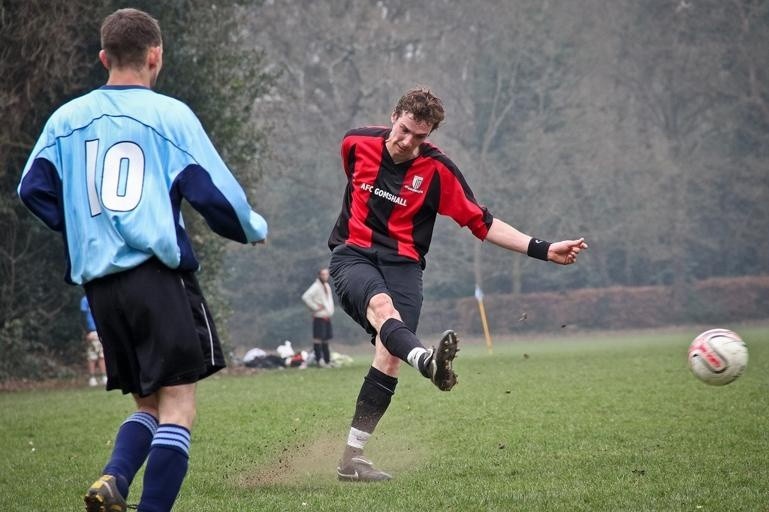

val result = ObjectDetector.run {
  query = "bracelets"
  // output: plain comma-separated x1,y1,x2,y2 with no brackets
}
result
527,237,553,261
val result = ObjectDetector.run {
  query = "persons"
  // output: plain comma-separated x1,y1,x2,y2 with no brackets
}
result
301,266,335,370
329,90,588,483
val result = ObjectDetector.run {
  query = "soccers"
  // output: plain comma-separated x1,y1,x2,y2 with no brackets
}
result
688,328,748,386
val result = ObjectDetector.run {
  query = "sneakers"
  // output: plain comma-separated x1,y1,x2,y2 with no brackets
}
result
89,376,108,386
418,330,460,391
337,455,392,481
84,475,127,512
